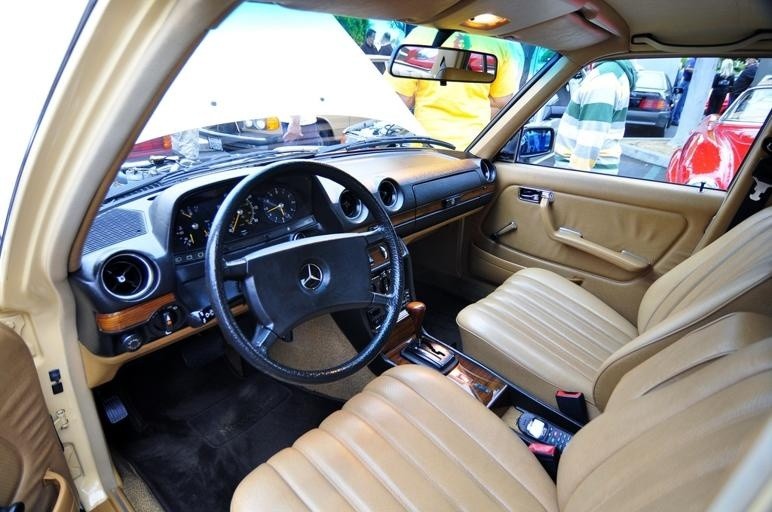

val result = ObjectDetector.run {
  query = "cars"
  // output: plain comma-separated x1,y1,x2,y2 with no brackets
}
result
535,58,731,133
120,134,176,169
392,46,495,77
0,0,771,512
194,55,424,154
663,76,772,193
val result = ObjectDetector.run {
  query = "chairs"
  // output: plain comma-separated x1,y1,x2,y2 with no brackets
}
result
230,334,772,512
452,204,771,418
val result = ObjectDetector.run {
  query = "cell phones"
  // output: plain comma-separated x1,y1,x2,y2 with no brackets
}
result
512,402,575,452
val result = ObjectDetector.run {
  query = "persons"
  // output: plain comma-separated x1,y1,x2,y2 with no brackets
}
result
554,60,638,175
383,25,518,150
361,29,378,66
378,33,394,74
727,57,760,112
705,58,735,116
671,57,696,126
279,110,324,146
171,129,199,160
